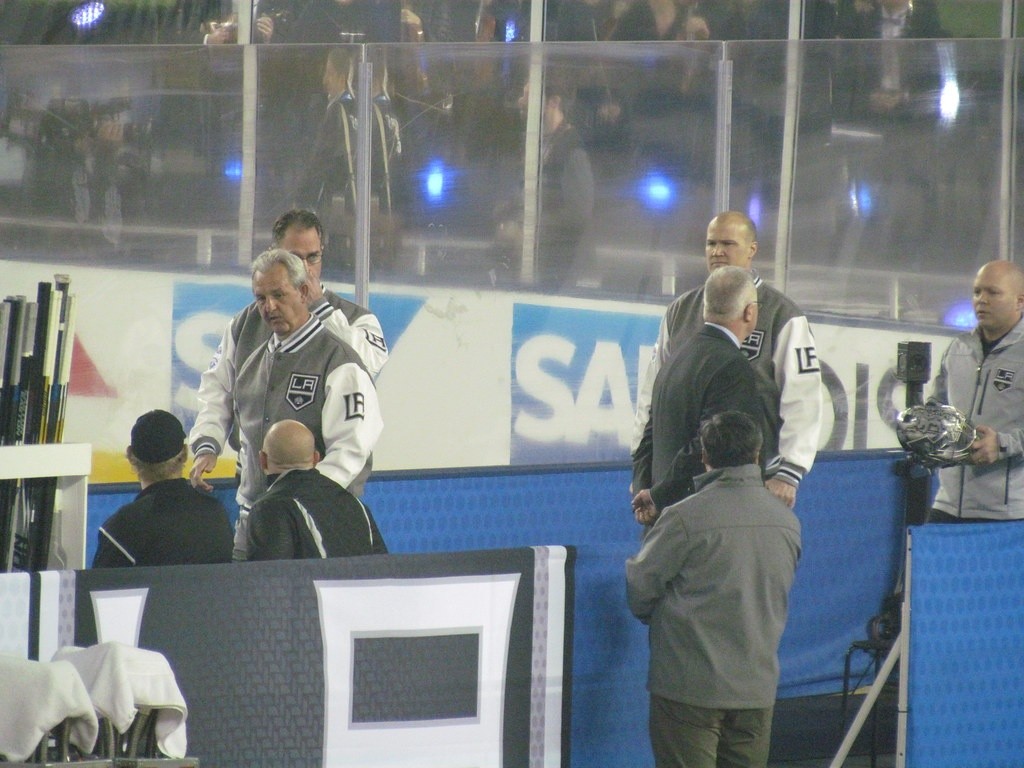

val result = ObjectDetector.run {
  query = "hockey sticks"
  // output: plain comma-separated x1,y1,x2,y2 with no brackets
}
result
0,707,158,763
0,273,79,566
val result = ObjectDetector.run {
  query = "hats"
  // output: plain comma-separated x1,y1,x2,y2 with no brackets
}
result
130,409,186,463
328,47,355,101
369,57,391,102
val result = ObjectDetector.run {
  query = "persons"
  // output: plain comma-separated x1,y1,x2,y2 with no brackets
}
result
631,266,762,528
625,412,802,768
190,210,388,560
924,259,1024,525
0,0,936,293
630,212,821,510
246,420,389,560
93,410,233,566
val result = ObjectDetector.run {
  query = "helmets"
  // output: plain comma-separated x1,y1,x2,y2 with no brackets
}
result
896,404,982,469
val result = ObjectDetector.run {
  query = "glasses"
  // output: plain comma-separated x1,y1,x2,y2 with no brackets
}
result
297,243,322,266
